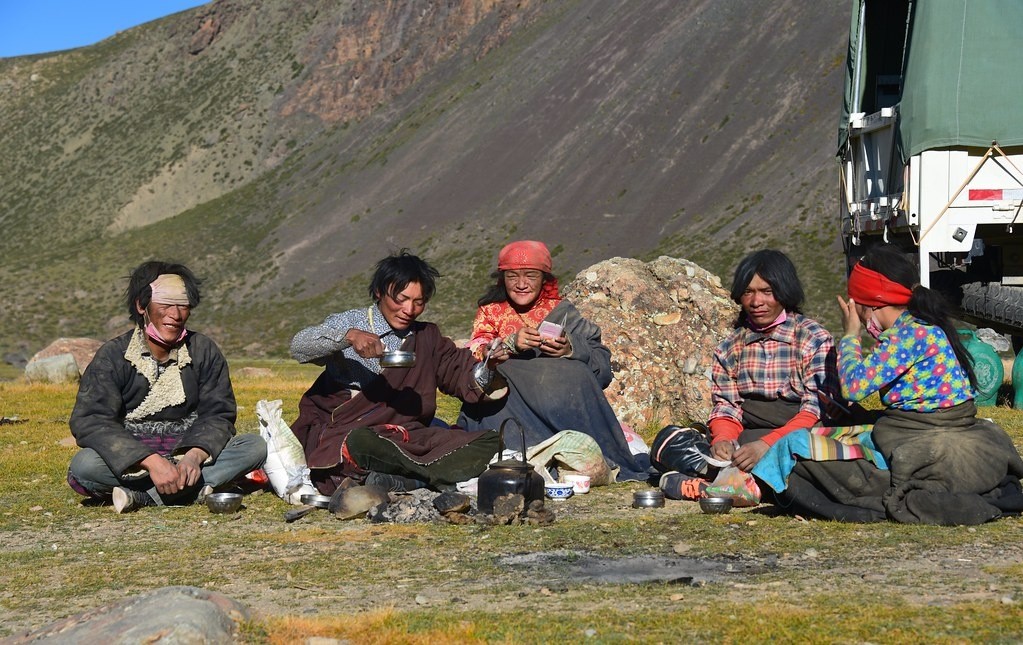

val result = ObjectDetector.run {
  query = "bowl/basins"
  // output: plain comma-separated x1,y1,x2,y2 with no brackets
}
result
380,351,417,368
564,475,591,495
205,492,243,514
633,490,665,508
544,483,574,501
699,497,734,514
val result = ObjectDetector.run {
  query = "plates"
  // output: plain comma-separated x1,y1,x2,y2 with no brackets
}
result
300,494,330,508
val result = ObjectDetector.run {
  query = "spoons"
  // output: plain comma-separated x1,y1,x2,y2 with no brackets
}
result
473,338,501,379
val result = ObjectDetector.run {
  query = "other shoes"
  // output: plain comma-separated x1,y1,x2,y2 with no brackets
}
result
366,472,406,493
113,485,145,515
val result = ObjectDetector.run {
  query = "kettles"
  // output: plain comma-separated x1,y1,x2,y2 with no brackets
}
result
477,417,545,514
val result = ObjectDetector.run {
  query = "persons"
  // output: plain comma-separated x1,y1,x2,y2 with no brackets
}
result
457,239,650,483
67,260,268,514
290,247,507,497
787,249,1023,523
652,250,854,500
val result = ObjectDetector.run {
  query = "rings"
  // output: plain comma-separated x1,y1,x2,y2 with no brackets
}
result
502,344,506,349
525,339,528,343
504,349,507,355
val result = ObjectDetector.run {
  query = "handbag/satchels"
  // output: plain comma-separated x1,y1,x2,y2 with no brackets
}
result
649,421,717,480
705,439,764,509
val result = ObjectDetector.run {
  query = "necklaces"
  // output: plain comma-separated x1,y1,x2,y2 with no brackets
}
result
368,307,407,346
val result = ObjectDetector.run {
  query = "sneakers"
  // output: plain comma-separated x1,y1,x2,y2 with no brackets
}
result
660,471,711,502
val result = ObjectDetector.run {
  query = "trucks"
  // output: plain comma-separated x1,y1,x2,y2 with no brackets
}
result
837,0,1023,356
